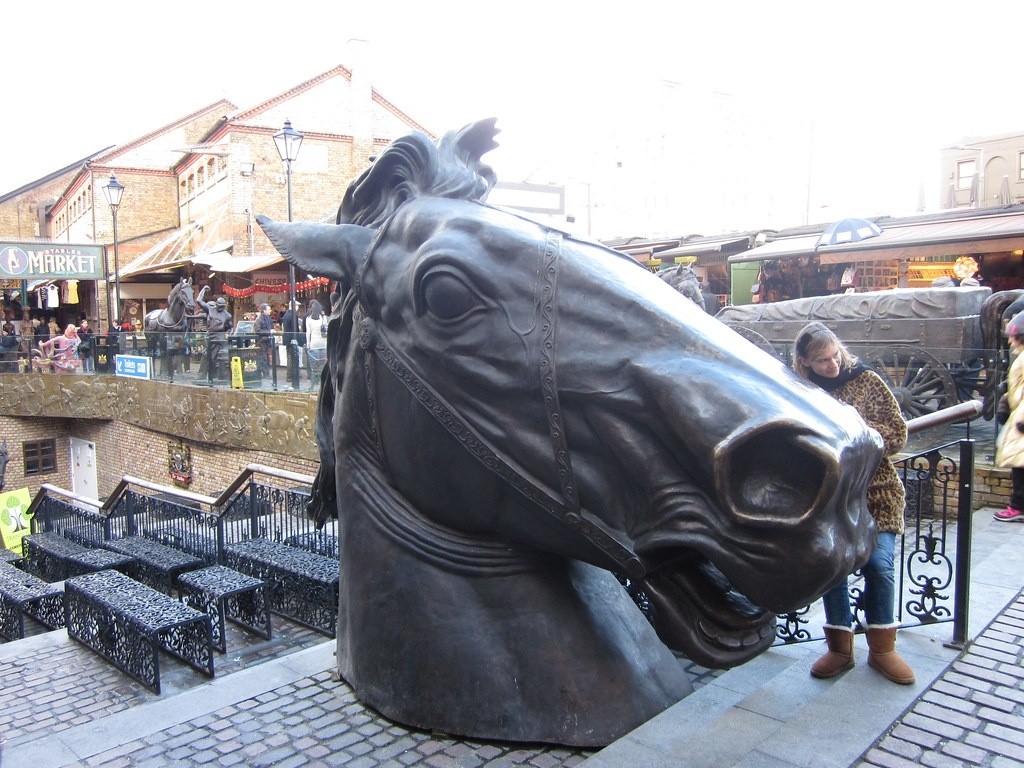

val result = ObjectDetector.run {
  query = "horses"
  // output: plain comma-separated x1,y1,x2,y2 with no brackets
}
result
145,276,196,380
254,114,885,747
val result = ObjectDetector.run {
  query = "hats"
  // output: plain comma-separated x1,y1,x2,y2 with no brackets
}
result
1004,309,1024,334
289,300,301,306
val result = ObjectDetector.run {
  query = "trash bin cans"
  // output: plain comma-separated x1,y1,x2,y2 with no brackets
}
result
227,334,262,389
93,334,120,374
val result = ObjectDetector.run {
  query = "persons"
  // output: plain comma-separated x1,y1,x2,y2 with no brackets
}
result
110,320,125,345
993,310,1024,520
77,320,95,373
792,320,915,683
254,304,274,378
17,309,37,372
701,282,720,316
4,316,15,335
278,299,329,391
186,286,232,380
933,277,981,403
33,313,80,374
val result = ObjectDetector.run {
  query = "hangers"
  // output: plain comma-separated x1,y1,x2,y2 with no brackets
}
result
66,280,80,282
35,285,45,291
46,284,59,289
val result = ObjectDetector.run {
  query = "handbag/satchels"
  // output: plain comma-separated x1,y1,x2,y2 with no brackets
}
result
751,266,779,304
80,340,91,349
321,315,328,338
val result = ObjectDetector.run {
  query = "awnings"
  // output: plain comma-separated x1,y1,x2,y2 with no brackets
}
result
210,256,284,272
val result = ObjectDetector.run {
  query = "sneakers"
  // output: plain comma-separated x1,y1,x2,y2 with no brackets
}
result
993,505,1024,522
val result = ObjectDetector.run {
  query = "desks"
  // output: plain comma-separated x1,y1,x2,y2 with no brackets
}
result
178,564,272,654
63,548,138,579
1,548,23,567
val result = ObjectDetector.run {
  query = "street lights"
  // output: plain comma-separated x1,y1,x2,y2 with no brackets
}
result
952,145,985,208
275,117,307,332
102,169,125,333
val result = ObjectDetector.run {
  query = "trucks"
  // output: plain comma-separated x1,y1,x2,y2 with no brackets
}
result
597,228,775,316
729,201,1024,306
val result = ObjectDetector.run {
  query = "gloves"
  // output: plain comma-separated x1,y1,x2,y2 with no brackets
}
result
996,412,1009,425
1017,422,1024,433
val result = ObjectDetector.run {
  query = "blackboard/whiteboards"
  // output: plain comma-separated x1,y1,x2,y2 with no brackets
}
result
233,321,255,336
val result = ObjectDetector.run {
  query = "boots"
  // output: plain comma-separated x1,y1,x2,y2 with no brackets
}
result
861,617,915,683
810,623,855,678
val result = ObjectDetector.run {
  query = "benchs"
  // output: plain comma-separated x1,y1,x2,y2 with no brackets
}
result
0,493,339,696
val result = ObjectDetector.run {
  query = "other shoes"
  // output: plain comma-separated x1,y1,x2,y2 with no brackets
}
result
259,375,272,379
174,370,177,372
287,375,301,382
186,370,190,372
